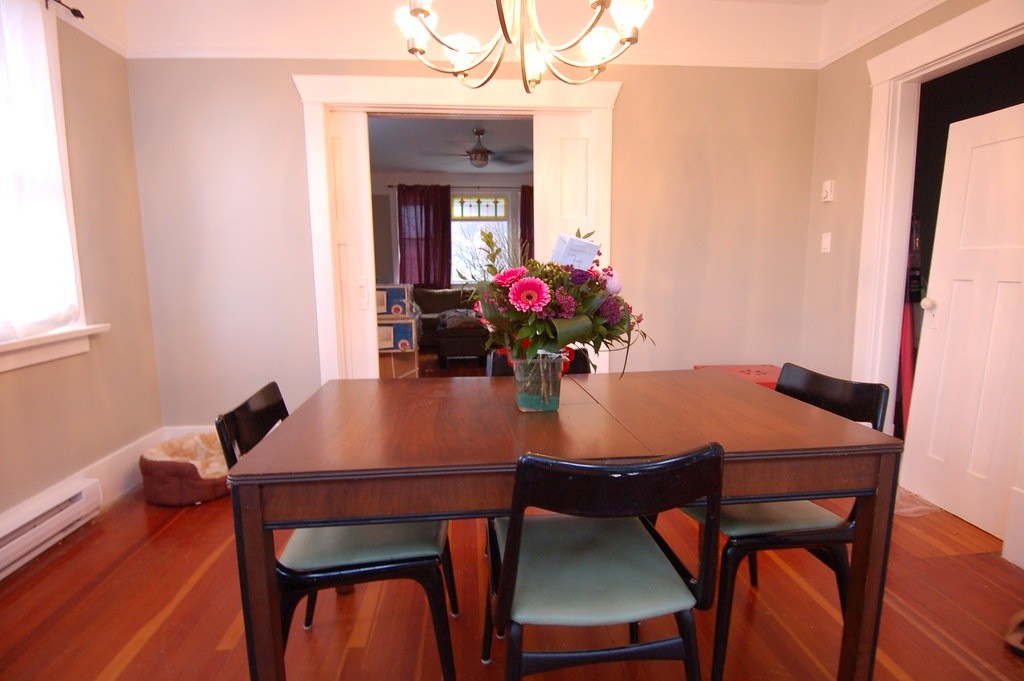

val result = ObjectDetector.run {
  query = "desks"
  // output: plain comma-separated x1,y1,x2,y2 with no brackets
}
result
224,368,905,681
379,344,419,378
691,365,782,390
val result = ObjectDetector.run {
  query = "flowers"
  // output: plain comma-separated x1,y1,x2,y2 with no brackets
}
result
456,228,656,406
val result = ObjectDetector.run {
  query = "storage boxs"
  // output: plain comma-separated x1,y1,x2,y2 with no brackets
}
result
376,283,423,350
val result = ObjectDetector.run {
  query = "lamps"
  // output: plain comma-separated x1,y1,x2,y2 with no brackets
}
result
392,0,654,95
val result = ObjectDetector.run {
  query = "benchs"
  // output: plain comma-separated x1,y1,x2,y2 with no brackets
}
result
436,322,489,369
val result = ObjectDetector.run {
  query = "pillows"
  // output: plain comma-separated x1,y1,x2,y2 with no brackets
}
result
436,309,483,329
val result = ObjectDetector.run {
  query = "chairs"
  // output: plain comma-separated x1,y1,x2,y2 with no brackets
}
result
214,381,459,681
479,349,724,681
647,363,891,680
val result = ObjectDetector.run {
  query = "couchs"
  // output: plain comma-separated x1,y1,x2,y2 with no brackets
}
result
414,288,485,346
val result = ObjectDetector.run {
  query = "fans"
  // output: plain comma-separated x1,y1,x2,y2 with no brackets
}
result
420,128,533,169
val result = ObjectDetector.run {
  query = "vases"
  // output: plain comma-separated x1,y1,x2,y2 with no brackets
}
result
507,352,570,412
